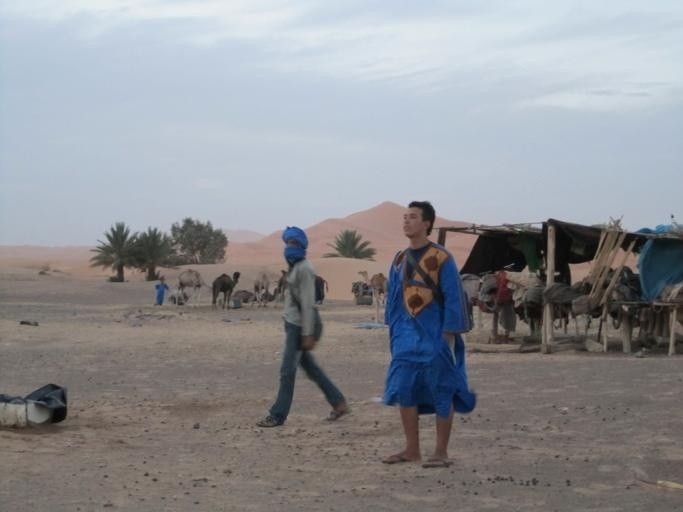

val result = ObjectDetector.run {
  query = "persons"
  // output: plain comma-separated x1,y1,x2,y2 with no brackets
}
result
152,275,169,306
256,225,354,428
379,199,477,468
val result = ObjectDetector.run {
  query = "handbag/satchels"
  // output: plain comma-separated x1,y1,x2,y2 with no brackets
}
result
462,289,474,331
315,274,328,305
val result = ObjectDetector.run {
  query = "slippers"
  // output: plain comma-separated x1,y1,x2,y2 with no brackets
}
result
422,458,454,467
381,454,407,463
257,416,282,427
322,406,351,421
